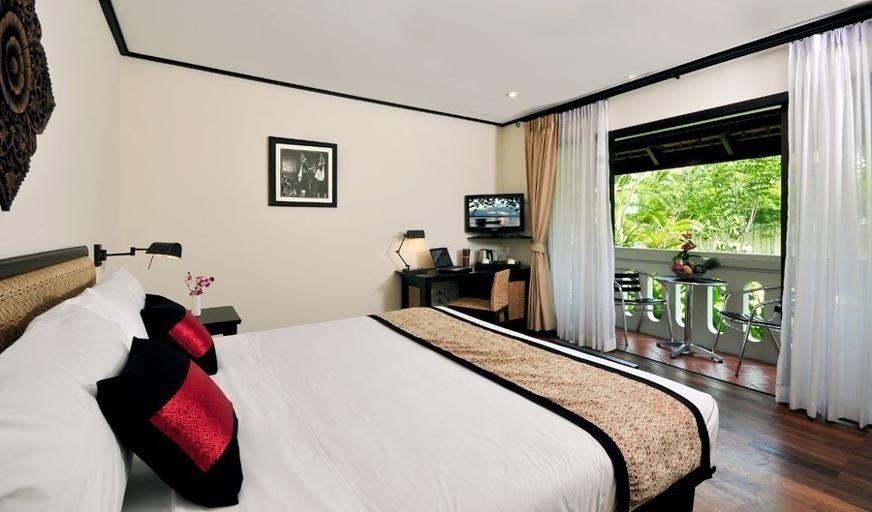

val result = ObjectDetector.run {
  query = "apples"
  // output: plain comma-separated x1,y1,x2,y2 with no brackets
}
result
681,265,692,272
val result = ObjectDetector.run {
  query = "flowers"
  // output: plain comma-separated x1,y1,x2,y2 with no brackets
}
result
672,232,700,261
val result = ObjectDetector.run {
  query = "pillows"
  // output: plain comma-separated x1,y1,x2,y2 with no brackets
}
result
140,293,218,377
0,268,149,512
95,335,242,509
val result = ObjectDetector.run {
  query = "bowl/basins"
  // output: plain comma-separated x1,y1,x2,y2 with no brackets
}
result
674,272,706,280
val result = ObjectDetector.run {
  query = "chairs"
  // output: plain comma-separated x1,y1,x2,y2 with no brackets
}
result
710,285,781,377
614,267,674,347
447,267,511,329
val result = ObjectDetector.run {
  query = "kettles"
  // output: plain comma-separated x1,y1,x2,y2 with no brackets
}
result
478,249,494,265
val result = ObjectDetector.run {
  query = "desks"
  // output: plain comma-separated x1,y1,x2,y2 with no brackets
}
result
395,264,530,329
654,276,728,363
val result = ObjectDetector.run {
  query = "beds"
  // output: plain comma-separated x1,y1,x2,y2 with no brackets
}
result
0,246,718,512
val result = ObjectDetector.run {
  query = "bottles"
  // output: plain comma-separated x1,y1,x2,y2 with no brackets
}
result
462,248,471,268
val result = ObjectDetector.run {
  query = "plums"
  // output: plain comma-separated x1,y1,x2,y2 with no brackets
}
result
671,263,682,271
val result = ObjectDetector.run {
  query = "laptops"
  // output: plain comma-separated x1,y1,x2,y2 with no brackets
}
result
429,247,473,273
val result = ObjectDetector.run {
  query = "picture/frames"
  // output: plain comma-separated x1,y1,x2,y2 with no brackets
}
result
269,136,337,207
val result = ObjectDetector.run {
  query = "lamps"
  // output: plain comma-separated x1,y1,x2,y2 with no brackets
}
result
395,231,427,275
94,242,181,270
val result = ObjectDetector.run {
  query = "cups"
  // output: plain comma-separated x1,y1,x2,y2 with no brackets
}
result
507,258,518,265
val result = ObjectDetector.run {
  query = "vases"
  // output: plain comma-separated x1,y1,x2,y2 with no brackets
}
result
182,270,216,317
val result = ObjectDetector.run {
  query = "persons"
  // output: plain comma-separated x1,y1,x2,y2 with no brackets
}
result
281,152,328,198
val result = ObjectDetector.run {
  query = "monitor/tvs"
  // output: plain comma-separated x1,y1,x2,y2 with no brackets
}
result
463,194,526,237
